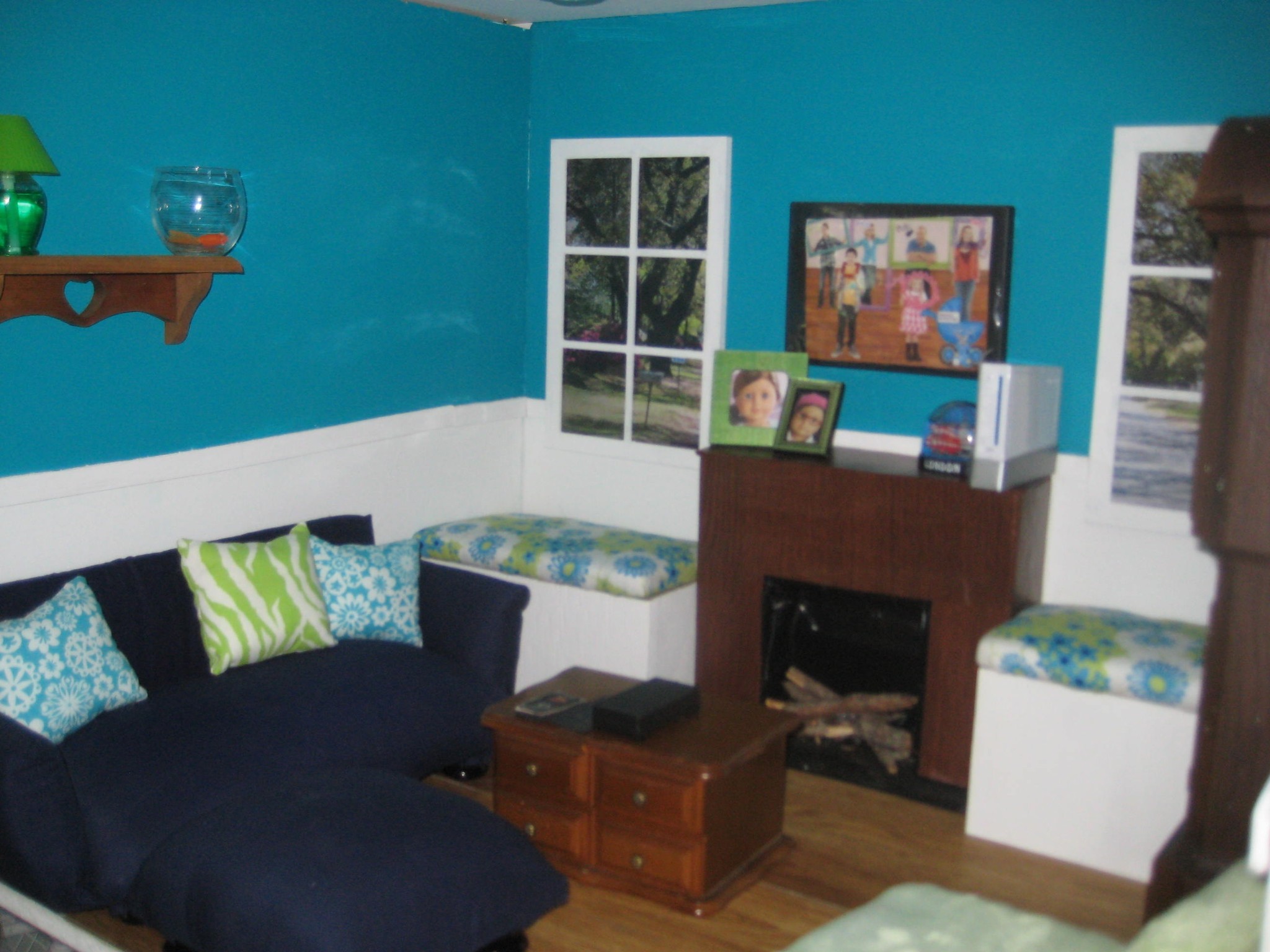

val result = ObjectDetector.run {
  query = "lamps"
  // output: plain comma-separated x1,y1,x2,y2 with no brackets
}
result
0,113,61,256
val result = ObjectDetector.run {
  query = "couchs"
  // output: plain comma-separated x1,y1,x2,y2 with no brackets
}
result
1,524,571,952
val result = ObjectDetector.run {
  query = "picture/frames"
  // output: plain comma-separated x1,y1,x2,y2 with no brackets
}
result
785,201,1015,381
771,376,845,457
708,349,809,448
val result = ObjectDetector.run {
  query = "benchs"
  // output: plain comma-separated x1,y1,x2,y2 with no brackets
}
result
962,602,1213,881
411,515,699,693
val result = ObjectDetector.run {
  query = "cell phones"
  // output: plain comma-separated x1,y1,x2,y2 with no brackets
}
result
514,689,585,718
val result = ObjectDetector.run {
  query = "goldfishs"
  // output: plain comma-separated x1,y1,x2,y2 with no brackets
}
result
167,230,230,248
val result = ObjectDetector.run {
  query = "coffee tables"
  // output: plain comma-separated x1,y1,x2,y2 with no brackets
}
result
478,665,803,917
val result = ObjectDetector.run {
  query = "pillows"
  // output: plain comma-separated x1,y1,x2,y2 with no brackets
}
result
307,534,425,648
177,521,338,675
0,575,149,747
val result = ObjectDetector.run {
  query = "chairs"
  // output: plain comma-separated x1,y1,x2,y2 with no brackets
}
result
779,847,1267,952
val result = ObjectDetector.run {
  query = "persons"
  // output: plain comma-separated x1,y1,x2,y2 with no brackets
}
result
728,367,781,429
785,392,827,443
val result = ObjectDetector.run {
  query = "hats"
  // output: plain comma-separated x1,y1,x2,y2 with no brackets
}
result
795,395,828,409
